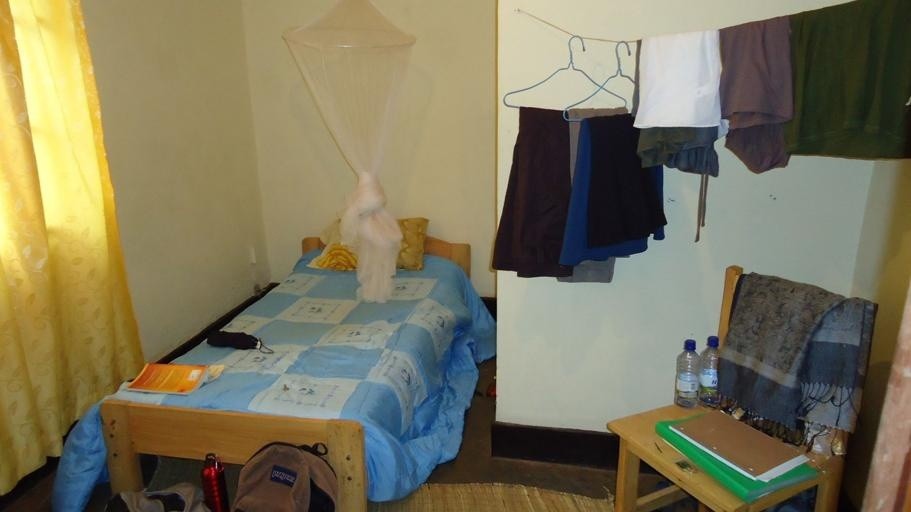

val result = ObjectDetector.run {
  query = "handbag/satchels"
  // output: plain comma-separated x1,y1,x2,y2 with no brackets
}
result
103,479,207,512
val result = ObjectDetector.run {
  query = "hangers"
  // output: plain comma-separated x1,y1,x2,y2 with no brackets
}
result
503,36,627,113
563,40,635,122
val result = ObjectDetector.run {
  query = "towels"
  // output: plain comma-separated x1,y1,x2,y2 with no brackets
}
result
634,31,722,128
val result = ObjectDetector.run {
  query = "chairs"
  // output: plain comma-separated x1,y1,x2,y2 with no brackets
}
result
606,265,878,512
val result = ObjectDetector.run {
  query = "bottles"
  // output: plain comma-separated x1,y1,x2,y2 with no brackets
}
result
201,451,229,511
700,335,722,408
674,338,703,405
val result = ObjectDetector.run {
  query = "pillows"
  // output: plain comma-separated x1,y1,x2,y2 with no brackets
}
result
306,218,428,271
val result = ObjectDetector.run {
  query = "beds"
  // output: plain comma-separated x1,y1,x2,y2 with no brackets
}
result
101,235,470,512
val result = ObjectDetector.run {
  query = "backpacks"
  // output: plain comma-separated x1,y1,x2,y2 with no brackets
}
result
230,440,341,512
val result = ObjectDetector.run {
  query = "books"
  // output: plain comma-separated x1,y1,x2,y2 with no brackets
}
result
125,362,225,396
